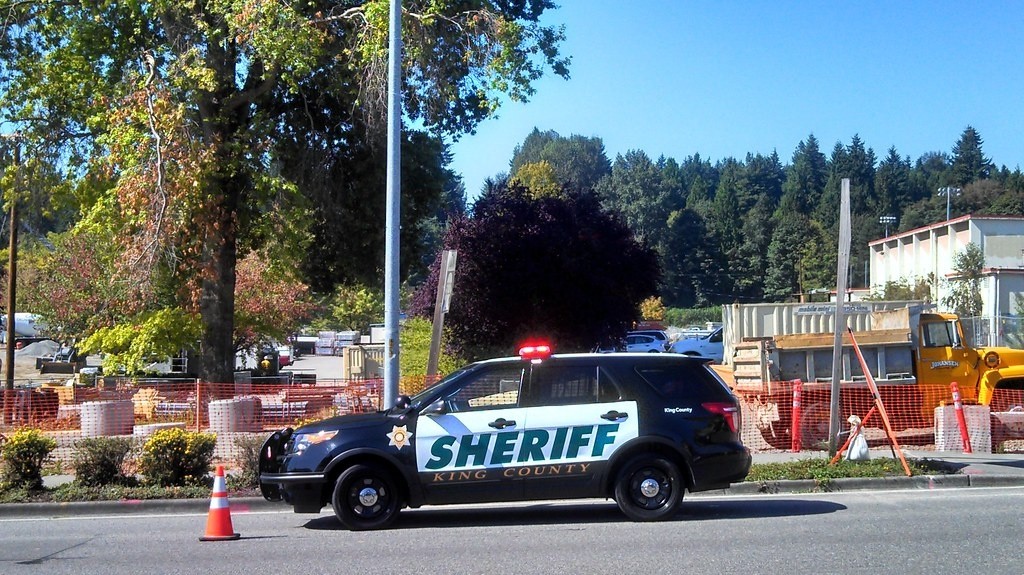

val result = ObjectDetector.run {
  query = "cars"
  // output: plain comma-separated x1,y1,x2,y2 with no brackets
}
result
589,330,670,352
668,323,724,364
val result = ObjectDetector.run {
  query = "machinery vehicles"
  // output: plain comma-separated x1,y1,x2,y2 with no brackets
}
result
0,312,75,349
36,333,89,374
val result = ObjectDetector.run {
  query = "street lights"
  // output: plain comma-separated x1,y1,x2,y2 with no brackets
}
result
879,216,897,238
937,185,962,220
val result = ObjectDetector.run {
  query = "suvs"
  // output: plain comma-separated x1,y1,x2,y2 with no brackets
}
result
258,346,752,531
235,333,299,371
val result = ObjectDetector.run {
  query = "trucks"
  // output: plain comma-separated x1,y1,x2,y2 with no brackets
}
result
731,312,1024,451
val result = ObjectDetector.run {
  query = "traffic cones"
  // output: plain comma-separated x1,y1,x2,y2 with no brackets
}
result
199,466,241,541
